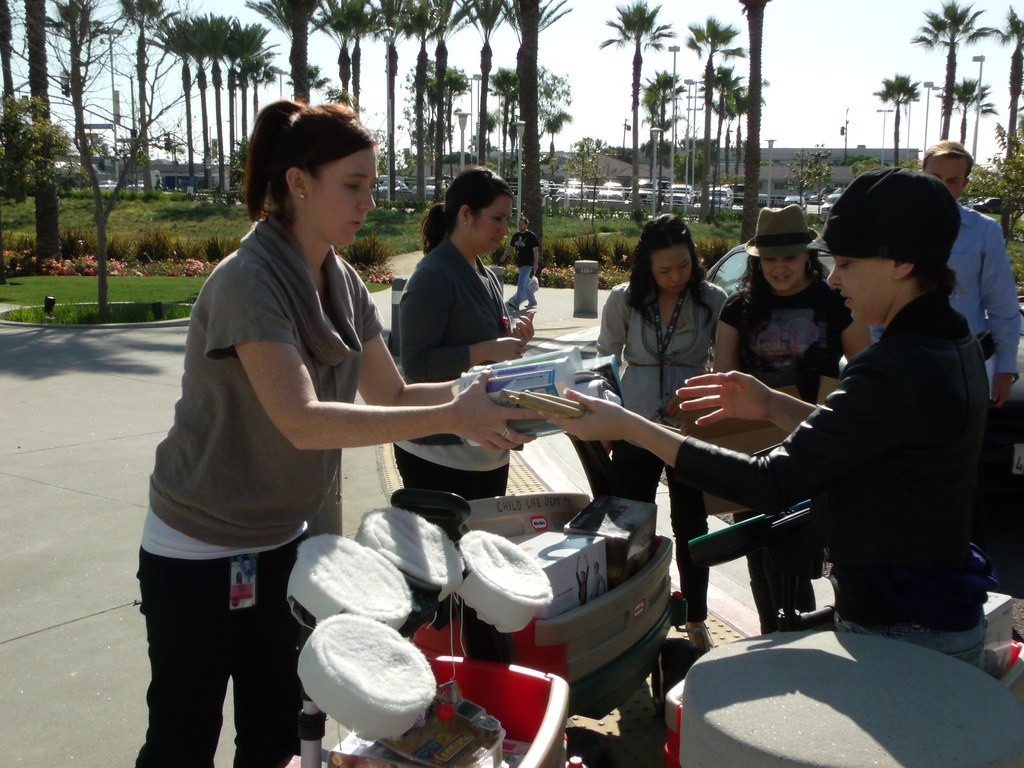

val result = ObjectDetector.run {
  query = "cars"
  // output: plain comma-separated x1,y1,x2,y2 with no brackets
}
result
629,179,660,203
969,197,1001,214
99,179,144,189
664,184,745,207
784,187,845,214
540,179,551,196
373,174,448,200
597,183,626,201
557,180,590,199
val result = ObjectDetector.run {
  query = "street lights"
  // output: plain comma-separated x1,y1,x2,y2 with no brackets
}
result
972,56,985,164
684,79,705,212
877,110,894,166
924,82,944,155
513,121,526,232
650,127,663,219
668,46,680,212
454,74,501,176
766,139,777,207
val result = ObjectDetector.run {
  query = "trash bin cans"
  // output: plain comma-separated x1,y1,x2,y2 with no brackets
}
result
488,265,504,291
573,260,598,318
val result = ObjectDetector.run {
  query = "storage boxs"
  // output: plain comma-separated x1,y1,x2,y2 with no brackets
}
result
282,378,1024,768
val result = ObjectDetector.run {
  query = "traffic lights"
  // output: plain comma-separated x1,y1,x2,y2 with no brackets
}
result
62,84,70,97
164,133,170,151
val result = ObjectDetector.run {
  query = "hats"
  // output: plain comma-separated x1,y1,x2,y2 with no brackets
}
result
804,170,962,266
743,204,819,259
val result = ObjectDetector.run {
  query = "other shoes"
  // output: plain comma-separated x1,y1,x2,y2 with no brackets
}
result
505,301,519,311
525,302,537,308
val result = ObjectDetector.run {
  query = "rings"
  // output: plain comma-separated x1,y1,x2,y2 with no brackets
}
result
501,428,513,439
520,335,525,341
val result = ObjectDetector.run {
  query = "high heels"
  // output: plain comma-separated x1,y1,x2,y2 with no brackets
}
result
682,623,713,654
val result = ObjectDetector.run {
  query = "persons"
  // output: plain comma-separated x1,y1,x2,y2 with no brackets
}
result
711,204,872,636
870,142,1020,569
501,216,538,311
391,165,536,500
134,99,546,768
539,167,990,675
596,213,728,652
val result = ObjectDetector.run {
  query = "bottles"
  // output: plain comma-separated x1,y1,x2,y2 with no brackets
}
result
565,756,588,768
